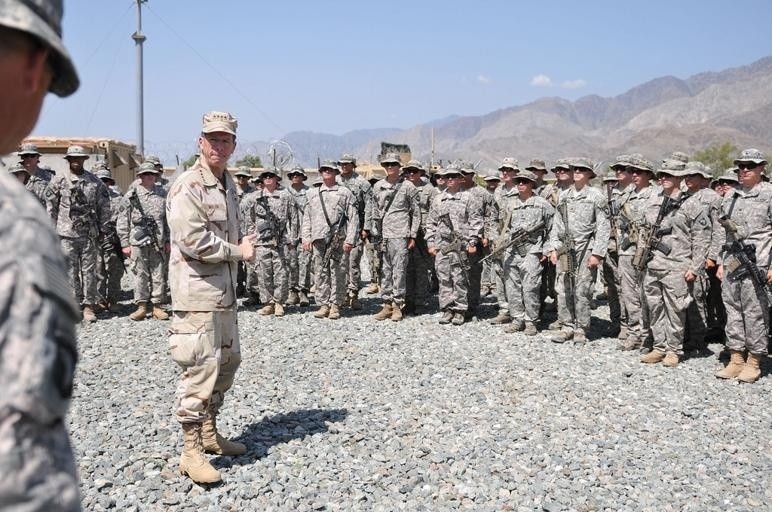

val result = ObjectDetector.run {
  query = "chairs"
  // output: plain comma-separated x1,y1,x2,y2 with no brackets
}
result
80,291,170,323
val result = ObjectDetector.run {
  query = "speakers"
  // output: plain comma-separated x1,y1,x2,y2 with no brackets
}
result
201,418,246,455
180,422,221,483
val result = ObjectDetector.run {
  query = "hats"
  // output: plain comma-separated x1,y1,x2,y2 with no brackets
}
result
201,111,238,137
1,0,79,97
6,143,165,186
233,148,771,190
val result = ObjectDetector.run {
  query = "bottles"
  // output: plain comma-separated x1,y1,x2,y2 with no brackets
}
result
6,143,165,186
201,111,238,137
1,0,79,97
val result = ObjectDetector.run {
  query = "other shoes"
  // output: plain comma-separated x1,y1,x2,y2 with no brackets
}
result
350,297,361,309
366,283,378,294
684,320,726,357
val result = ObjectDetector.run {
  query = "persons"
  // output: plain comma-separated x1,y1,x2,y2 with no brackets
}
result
162,108,261,483
0,142,771,383
0,0,89,511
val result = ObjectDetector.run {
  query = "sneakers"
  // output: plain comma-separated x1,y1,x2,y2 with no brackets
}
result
285,292,299,304
617,339,645,352
551,330,572,343
260,305,274,315
524,323,537,335
439,311,453,323
242,297,261,306
505,322,522,333
596,291,609,300
640,348,665,362
275,304,284,317
548,320,560,330
451,310,465,324
662,353,680,366
573,331,586,343
341,297,350,308
299,292,309,306
329,304,340,319
315,305,330,318
619,328,627,339
488,312,512,323
80,291,170,323
604,322,620,336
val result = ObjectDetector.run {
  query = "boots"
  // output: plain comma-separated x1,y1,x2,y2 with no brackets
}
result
391,301,402,321
375,304,392,320
716,350,749,378
738,352,760,381
201,418,246,455
180,422,221,483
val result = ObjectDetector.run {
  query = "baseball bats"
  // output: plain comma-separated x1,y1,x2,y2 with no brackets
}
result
255,196,280,244
366,218,388,285
69,186,100,239
439,212,471,286
316,212,349,282
633,195,679,287
128,194,160,253
478,221,547,263
554,202,577,296
718,215,772,306
620,203,638,251
